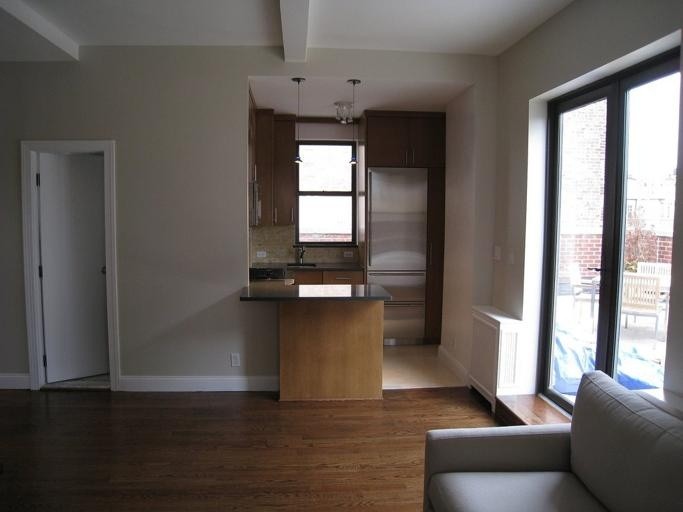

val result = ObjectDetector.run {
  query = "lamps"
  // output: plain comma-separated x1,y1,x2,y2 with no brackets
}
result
334,101,353,124
292,78,306,164
347,79,361,165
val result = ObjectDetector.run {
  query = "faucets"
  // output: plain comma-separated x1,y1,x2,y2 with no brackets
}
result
292,244,306,264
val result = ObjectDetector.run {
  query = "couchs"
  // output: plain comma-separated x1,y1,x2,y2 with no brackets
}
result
424,370,682,512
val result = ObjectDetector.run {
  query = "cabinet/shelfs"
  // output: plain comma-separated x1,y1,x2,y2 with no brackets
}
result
288,271,364,285
248,85,295,228
358,109,445,167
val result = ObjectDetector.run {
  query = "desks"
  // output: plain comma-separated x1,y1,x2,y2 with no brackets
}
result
591,275,671,317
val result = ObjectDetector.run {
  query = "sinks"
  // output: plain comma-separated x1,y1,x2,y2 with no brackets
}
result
287,262,317,267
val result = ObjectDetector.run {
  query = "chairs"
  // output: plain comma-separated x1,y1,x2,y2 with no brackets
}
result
635,262,671,321
569,260,599,316
621,272,660,336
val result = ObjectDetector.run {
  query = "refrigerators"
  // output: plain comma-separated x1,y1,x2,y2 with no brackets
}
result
366,166,427,341
